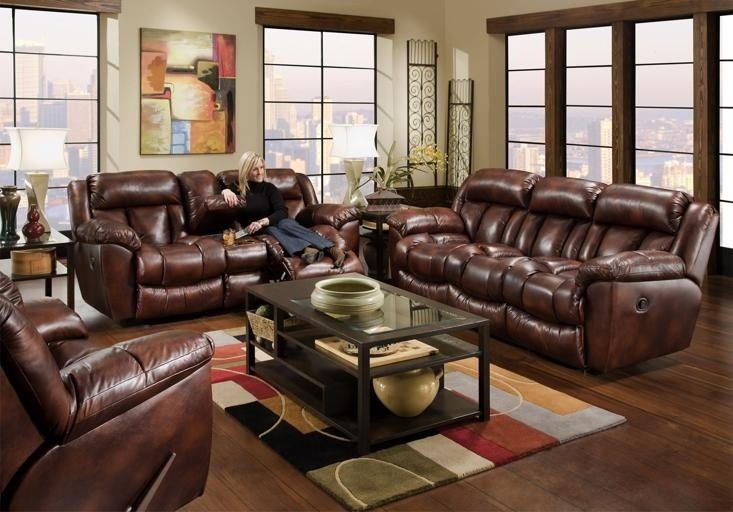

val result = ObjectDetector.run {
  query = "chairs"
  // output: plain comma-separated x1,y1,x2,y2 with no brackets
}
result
0,272,213,510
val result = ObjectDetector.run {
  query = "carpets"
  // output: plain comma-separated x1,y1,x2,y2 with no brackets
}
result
202,325,627,511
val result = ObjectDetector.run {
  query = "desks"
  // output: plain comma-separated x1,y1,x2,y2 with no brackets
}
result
361,204,420,280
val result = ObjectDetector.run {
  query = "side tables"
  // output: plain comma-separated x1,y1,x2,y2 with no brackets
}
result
0,227,74,309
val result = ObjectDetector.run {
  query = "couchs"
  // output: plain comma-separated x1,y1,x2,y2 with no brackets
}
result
67,169,364,326
386,168,720,373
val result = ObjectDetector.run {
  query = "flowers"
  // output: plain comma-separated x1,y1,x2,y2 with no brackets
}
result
352,139,447,190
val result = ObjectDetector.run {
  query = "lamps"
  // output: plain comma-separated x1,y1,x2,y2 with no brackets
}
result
329,123,378,207
5,127,69,234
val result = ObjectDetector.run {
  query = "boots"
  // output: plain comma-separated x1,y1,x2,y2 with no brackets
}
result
301,246,325,264
325,245,350,268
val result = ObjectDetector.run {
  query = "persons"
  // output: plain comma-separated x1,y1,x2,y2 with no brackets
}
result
217,152,346,269
